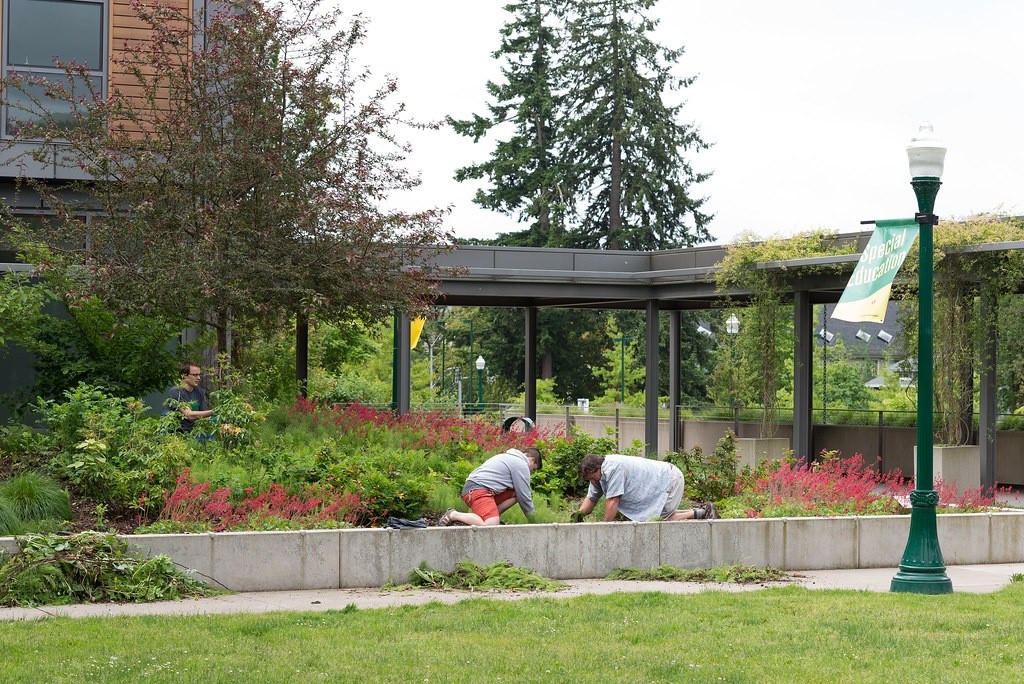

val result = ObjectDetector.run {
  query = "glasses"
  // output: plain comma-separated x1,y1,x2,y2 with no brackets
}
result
533,460,537,471
189,373,202,377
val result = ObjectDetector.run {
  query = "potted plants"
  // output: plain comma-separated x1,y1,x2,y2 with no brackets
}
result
705,205,1024,504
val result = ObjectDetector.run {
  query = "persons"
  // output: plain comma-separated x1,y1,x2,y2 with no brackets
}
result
438,448,543,527
157,361,214,445
572,455,721,522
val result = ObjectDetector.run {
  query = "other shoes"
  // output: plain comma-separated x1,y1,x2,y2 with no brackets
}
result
700,502,721,519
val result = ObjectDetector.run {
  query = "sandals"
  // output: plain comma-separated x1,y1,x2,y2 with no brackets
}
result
438,508,455,527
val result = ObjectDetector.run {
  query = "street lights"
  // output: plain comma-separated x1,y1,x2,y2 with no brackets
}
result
476,355,492,413
726,312,741,438
861,119,956,593
612,335,634,402
436,321,447,391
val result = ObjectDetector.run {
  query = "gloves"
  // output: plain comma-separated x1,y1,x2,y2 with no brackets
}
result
570,511,583,522
